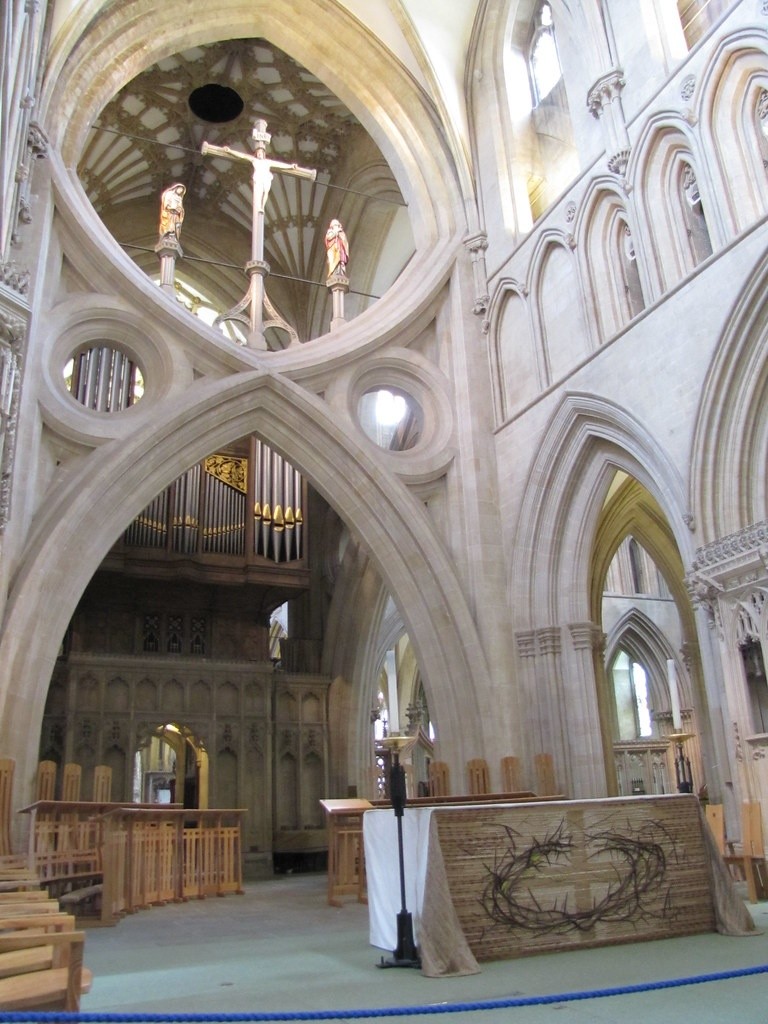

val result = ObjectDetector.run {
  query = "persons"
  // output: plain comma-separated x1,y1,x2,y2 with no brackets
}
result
325,219,349,277
159,184,186,241
224,146,298,214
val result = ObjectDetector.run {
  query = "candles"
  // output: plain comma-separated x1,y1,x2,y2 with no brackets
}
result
386,649,399,732
666,659,682,729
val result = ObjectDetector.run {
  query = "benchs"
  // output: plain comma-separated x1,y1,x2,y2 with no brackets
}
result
0,848,104,1013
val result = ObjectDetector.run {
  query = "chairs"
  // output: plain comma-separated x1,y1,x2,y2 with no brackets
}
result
705,804,733,881
722,802,768,904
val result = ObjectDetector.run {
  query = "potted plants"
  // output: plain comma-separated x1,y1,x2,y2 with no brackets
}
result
154,778,171,804
